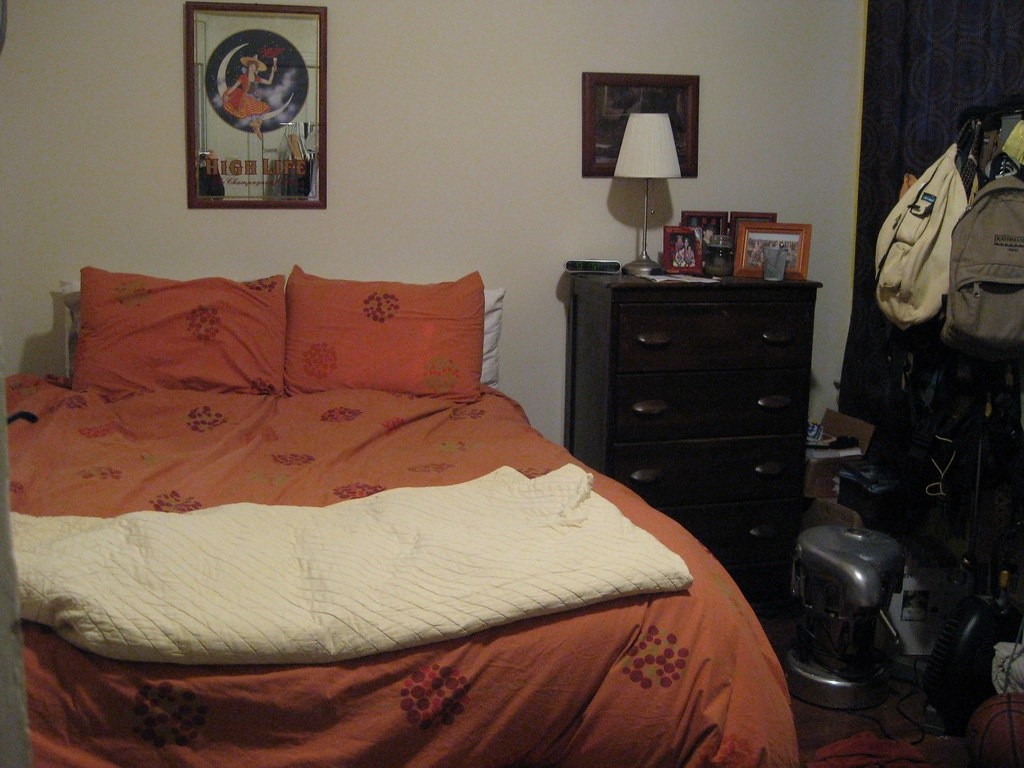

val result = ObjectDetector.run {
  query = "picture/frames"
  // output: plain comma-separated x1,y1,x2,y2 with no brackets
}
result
663,225,703,275
185,1,327,210
733,220,813,281
680,210,728,269
729,211,778,254
581,72,701,177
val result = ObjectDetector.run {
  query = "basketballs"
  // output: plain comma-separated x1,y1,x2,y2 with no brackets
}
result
963,693,1024,768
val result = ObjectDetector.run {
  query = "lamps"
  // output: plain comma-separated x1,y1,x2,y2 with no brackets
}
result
614,112,682,276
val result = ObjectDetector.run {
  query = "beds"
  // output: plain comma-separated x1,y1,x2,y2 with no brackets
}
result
0,264,800,768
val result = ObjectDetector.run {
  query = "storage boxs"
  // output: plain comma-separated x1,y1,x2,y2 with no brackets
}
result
802,408,876,541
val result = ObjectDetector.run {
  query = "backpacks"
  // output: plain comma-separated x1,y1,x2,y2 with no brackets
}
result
938,175,1024,360
873,140,969,330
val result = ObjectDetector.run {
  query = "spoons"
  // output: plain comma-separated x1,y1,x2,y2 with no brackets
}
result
769,243,785,277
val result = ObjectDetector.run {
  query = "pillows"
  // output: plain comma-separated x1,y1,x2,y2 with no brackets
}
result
285,265,485,404
71,266,286,397
479,289,507,389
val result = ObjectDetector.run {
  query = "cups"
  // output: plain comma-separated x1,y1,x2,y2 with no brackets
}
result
763,247,788,281
705,236,733,277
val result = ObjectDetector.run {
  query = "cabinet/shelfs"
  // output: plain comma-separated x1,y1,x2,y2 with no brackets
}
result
563,271,824,616
